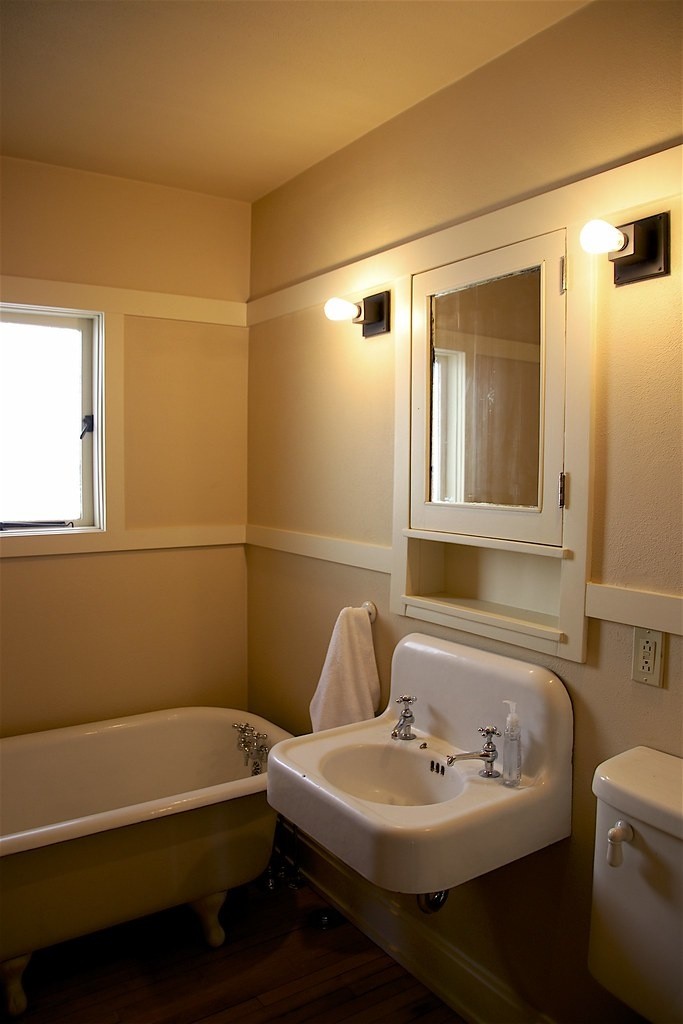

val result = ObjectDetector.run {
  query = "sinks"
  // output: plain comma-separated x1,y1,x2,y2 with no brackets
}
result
314,739,467,807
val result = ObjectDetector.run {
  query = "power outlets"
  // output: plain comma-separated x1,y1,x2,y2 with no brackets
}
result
630,626,667,688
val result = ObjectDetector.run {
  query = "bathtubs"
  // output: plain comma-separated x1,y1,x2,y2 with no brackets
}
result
0,705,299,965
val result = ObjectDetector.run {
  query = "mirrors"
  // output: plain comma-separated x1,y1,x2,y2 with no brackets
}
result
424,259,547,512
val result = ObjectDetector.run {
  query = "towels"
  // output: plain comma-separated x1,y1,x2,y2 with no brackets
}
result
308,606,382,733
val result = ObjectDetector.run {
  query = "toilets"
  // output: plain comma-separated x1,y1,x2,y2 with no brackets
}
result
587,744,683,1024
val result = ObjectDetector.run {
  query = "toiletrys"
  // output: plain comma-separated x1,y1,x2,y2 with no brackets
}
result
502,699,522,787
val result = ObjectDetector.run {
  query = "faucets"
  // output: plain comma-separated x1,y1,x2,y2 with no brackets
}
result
390,694,418,740
446,725,502,778
